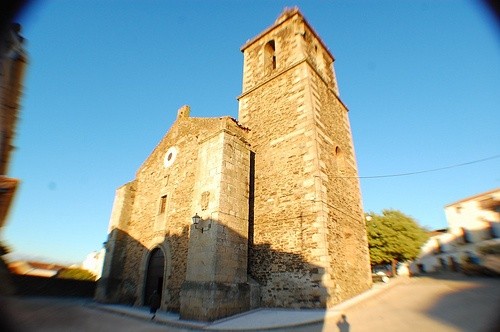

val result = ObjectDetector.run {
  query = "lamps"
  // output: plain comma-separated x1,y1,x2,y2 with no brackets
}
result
365,216,372,226
193,213,201,230
103,242,108,248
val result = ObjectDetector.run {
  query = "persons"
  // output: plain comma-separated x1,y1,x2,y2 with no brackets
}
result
148,290,162,320
